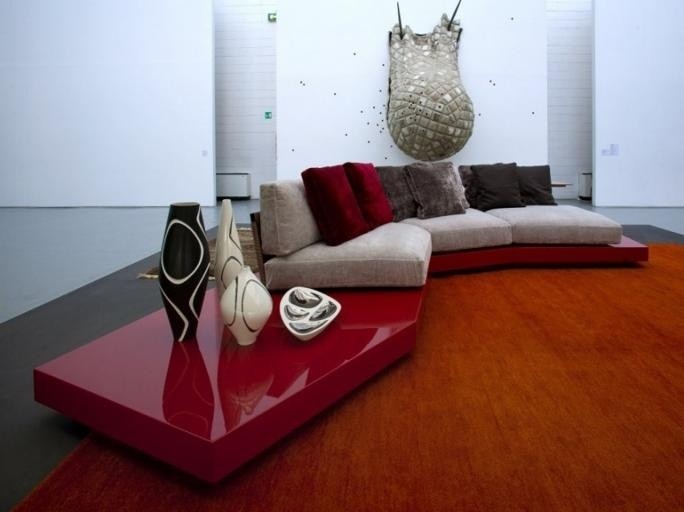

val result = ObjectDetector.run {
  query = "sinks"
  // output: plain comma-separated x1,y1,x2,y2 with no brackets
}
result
249,181,647,289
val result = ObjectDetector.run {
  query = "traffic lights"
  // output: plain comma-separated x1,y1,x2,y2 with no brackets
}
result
302,162,557,245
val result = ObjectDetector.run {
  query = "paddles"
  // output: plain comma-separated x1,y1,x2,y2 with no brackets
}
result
1,242,684,511
138,227,259,281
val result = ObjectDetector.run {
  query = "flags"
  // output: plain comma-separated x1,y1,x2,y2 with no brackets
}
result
159,198,273,347
